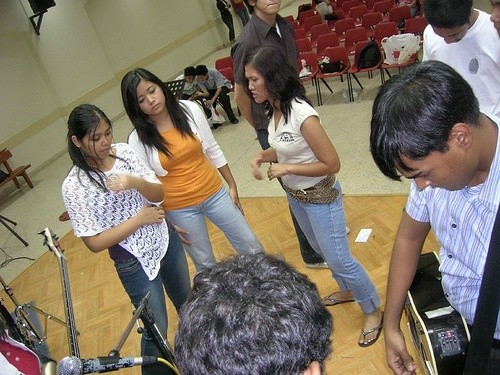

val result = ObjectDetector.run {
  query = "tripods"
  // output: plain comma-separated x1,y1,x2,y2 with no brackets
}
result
0,277,80,355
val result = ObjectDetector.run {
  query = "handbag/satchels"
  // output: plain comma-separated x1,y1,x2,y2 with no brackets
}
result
381,33,421,65
318,59,345,72
356,36,381,69
208,104,226,123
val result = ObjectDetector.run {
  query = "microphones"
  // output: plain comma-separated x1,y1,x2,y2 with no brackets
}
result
57,355,157,375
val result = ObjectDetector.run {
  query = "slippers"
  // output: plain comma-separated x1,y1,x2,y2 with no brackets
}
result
358,311,384,347
320,291,355,305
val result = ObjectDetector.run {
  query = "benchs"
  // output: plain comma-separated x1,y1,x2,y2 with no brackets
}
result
0,147,33,188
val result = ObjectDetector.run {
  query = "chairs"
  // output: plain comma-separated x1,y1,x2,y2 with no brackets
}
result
215,0,428,117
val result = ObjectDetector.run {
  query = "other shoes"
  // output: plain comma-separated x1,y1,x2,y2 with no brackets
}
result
230,118,238,124
210,123,222,130
304,261,329,268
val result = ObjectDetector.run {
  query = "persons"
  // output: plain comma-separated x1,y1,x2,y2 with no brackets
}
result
215,0,331,268
370,0,500,375
175,252,334,375
62,104,191,375
121,65,265,273
243,43,384,348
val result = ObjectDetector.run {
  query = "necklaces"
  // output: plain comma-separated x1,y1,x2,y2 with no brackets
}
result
100,152,125,192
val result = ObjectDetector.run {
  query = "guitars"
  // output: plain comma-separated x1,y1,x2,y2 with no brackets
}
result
36,225,84,374
395,248,471,375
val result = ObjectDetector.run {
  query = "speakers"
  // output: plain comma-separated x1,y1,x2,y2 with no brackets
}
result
28,0,56,14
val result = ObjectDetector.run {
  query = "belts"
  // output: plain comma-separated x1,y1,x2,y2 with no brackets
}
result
278,173,339,204
